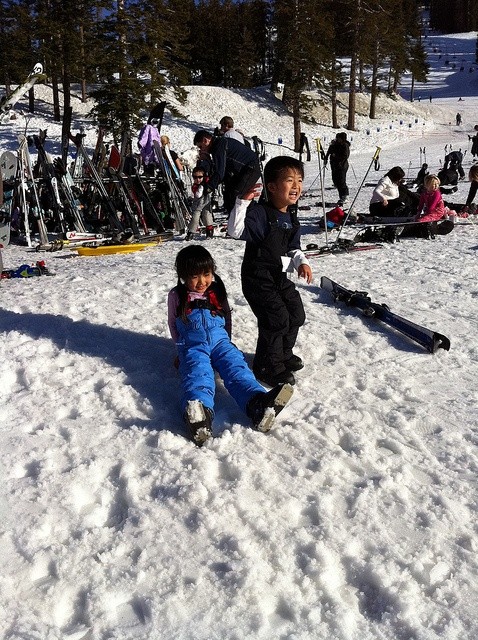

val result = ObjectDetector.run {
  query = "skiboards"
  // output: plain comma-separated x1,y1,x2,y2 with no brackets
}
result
33,135,71,240
153,143,187,233
69,132,124,232
320,276,451,354
18,134,49,247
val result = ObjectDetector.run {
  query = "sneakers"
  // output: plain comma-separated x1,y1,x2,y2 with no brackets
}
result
282,355,304,371
253,369,295,389
247,382,294,434
184,399,214,449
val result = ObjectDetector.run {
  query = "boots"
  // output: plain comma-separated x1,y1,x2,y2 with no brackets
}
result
206,229,213,240
186,232,193,241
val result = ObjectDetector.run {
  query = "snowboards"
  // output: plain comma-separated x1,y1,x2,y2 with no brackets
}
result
95,146,121,220
165,145,181,179
354,220,454,242
66,232,105,240
78,243,158,256
0,151,17,248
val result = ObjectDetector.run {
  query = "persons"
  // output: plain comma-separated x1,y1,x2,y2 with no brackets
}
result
472,125,478,156
240,155,312,389
458,97,461,101
161,136,183,177
370,167,405,218
417,164,458,194
235,128,255,152
462,163,478,215
323,132,350,206
193,130,263,239
168,245,293,450
415,174,444,223
443,151,465,180
220,116,244,144
185,167,215,241
456,113,461,125
418,96,421,102
430,96,432,102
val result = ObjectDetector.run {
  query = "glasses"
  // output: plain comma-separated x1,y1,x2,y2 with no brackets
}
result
193,175,203,179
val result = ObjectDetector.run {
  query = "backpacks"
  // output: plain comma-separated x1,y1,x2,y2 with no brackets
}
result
239,132,268,160
